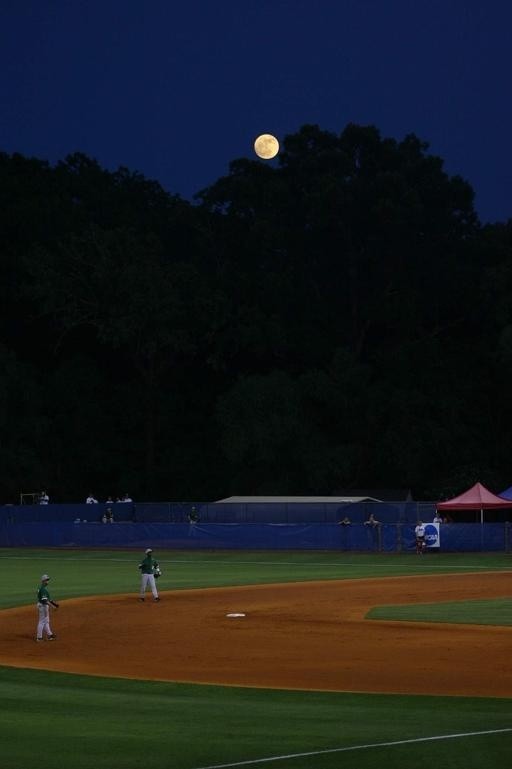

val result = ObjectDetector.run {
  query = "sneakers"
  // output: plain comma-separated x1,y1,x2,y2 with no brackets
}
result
139,598,144,601
36,635,56,641
154,598,160,602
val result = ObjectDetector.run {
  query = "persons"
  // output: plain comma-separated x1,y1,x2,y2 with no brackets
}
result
137,548,163,603
102,507,114,524
434,512,444,523
339,516,352,525
443,517,449,523
362,513,384,525
106,492,132,504
188,505,200,525
38,491,49,505
85,493,98,504
415,520,426,556
35,574,60,643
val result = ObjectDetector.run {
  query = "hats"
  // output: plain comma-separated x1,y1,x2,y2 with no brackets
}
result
42,574,50,581
145,549,152,554
